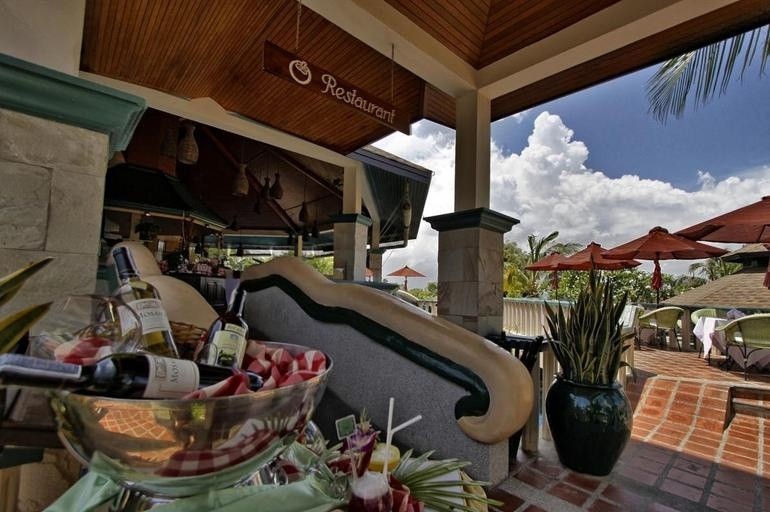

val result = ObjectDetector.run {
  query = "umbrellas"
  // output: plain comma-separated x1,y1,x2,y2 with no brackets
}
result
386,265,427,291
524,196,770,345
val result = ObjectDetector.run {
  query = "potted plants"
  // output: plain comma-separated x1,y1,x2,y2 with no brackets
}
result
539,252,638,478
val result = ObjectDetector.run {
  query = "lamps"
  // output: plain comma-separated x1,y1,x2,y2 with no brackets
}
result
135,214,158,244
177,122,200,165
231,138,321,243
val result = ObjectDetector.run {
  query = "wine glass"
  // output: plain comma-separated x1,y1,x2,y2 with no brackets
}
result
278,420,350,500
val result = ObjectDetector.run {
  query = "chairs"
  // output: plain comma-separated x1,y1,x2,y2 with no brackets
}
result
639,306,684,352
715,313,770,381
691,308,717,356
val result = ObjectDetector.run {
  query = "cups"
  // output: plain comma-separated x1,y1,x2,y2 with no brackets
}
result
345,474,394,512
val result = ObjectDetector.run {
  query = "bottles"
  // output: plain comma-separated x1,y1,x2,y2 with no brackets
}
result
1,248,263,398
